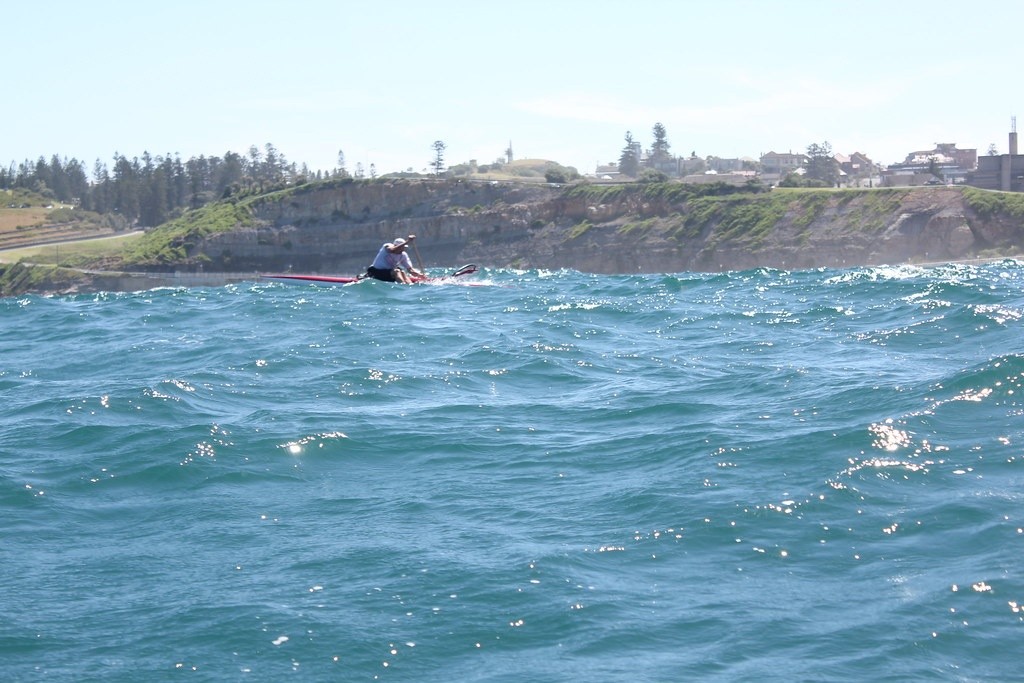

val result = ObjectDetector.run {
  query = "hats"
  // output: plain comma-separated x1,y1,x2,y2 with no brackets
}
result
393,237,409,248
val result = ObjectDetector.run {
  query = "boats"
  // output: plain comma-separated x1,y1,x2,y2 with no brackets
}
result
258,262,478,289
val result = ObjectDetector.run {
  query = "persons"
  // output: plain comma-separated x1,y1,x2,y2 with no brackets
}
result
368,234,427,285
